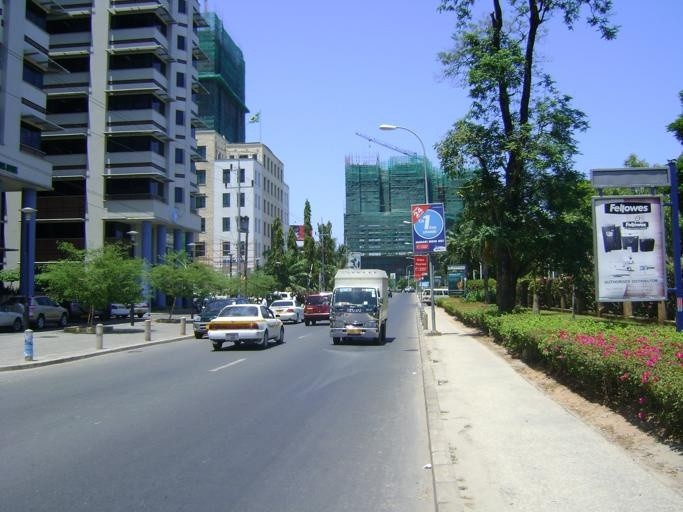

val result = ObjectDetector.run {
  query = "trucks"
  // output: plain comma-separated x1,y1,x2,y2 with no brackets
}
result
330,269,390,344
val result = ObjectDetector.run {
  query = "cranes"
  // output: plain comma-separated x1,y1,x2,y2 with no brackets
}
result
354,131,427,171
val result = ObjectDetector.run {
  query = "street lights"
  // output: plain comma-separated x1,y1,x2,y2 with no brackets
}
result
127,231,139,324
225,180,257,298
20,208,38,326
381,125,436,330
315,231,331,292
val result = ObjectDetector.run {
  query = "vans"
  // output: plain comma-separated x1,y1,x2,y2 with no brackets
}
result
421,289,449,305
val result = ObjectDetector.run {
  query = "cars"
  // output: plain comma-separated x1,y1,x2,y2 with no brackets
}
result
93,299,148,319
2,295,82,330
195,291,332,349
396,285,413,293
